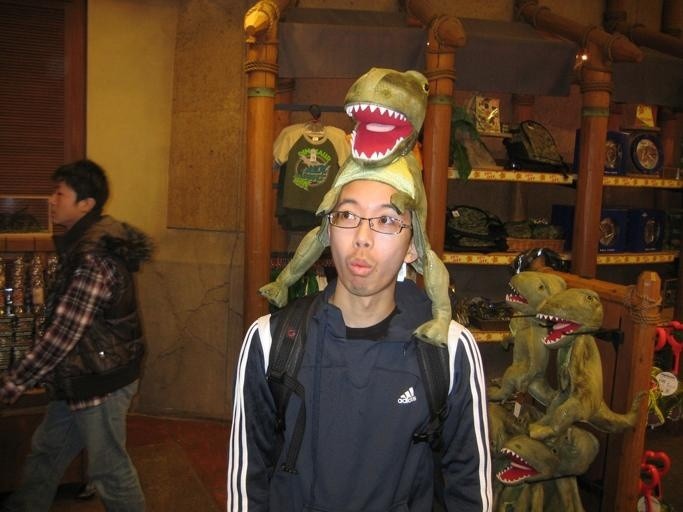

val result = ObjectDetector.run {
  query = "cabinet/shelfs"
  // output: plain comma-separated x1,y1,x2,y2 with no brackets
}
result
245,0,683,345
1,194,66,417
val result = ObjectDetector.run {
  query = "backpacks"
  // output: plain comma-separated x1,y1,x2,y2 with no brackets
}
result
503,120,566,172
446,206,509,253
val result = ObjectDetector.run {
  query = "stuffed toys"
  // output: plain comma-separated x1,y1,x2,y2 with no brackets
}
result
520,287,651,441
481,394,548,448
254,66,457,352
490,426,603,511
476,271,569,406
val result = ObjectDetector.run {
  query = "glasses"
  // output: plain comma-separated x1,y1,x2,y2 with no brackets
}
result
327,210,411,235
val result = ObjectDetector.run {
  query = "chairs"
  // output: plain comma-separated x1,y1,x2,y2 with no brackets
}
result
532,255,662,512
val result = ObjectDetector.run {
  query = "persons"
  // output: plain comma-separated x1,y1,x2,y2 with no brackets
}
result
220,157,500,509
0,157,161,510
